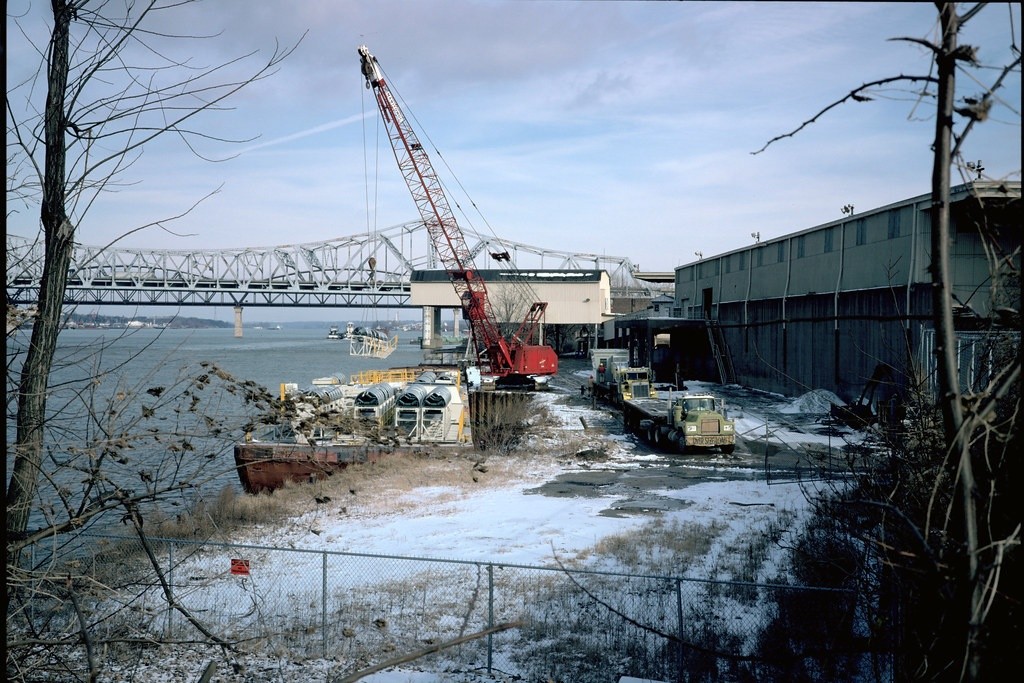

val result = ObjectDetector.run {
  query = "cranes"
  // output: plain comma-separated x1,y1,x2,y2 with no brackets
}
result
356,43,558,392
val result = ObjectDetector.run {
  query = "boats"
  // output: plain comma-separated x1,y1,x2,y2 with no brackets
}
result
327,321,354,341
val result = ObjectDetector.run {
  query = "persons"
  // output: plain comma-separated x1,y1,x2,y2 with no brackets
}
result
598,363,606,384
697,401,708,410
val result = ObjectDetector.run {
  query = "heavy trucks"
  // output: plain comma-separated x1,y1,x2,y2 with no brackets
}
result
578,348,652,410
624,387,737,455
